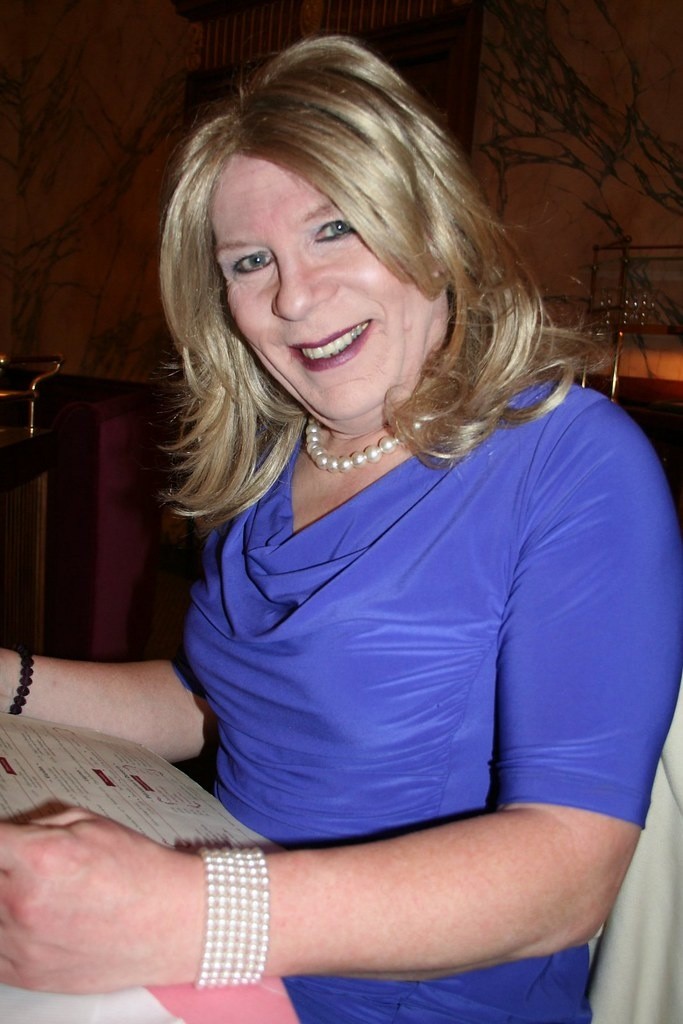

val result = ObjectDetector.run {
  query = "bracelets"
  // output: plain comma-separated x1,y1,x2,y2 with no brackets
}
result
194,845,270,990
8,650,34,716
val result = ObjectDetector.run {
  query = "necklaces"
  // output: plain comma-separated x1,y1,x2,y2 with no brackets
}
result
305,413,433,472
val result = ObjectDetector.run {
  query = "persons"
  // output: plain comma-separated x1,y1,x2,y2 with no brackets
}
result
0,35,683,1023
